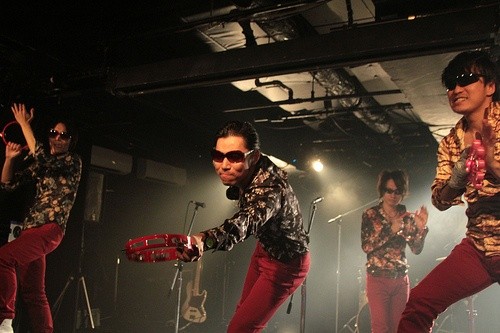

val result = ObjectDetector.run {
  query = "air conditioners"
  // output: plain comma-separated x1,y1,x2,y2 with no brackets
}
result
87,145,132,175
137,157,186,187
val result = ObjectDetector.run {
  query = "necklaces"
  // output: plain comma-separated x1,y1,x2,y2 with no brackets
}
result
387,210,398,218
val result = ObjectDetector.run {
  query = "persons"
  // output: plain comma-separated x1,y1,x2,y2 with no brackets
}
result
361,50,500,332
190,121,310,333
0,103,82,333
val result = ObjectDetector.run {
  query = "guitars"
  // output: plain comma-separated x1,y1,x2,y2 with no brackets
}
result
179,257,207,323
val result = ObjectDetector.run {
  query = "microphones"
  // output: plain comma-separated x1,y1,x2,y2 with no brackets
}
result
312,197,324,204
191,201,206,208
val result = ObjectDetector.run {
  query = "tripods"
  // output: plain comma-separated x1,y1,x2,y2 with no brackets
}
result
51,225,96,333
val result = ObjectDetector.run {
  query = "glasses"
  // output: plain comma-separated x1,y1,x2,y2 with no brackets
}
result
49,129,73,140
211,147,254,163
444,73,486,90
384,188,401,194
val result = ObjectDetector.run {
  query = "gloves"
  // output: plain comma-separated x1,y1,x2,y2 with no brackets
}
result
177,233,205,263
448,147,477,190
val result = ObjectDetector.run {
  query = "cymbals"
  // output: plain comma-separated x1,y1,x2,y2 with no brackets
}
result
397,210,428,242
435,257,447,261
0,121,29,151
465,140,486,191
119,234,200,263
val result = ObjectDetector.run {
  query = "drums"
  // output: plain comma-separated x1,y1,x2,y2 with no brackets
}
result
356,302,371,333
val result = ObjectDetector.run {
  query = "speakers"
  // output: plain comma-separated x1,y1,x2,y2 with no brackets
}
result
71,173,105,224
